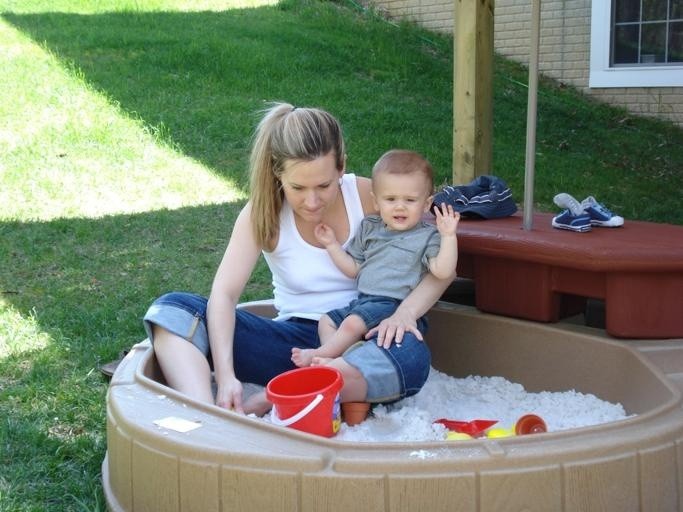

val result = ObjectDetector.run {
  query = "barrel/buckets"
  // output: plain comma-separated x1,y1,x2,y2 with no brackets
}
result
265,365,346,439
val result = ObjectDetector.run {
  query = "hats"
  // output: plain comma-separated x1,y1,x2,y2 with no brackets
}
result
430,174,519,221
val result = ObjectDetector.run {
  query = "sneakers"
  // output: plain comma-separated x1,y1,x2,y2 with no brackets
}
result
583,203,624,228
552,209,592,233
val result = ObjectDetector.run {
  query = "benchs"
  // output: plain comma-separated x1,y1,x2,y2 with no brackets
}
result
421,209,682,338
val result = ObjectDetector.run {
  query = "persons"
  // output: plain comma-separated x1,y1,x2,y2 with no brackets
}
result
142,101,429,419
288,148,460,368
